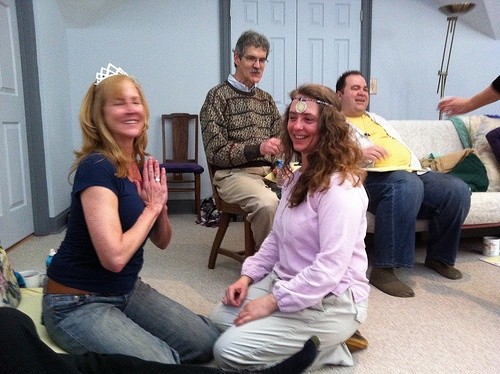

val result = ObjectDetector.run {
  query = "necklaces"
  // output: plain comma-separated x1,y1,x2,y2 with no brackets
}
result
348,116,370,136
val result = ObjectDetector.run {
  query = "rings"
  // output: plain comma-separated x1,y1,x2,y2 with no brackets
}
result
154,175,161,182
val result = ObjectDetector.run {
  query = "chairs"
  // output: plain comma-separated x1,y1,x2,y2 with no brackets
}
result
159,112,204,216
206,159,281,270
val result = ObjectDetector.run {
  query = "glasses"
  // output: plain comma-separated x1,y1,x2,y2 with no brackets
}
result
240,54,269,64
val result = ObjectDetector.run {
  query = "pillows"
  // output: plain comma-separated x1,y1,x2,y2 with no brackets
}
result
475,115,500,152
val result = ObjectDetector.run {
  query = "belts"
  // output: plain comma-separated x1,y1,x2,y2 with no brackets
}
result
44,277,99,294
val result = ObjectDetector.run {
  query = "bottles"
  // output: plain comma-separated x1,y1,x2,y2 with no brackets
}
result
46,248,56,267
276,159,286,198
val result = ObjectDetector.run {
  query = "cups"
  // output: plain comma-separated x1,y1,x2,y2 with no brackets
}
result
483,236,500,257
19,270,45,288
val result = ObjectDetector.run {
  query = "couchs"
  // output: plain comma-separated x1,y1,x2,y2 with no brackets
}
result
366,113,500,236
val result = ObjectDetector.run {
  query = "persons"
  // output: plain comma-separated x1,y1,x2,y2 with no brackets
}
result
41,63,220,365
336,70,472,298
207,84,369,371
199,30,292,252
435,75,500,116
0,306,320,374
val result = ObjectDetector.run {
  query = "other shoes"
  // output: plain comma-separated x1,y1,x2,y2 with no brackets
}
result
345,333,368,353
